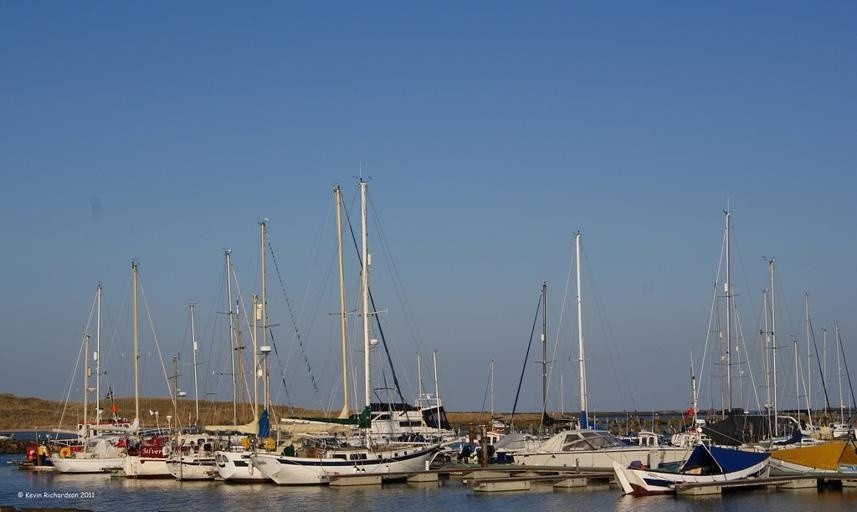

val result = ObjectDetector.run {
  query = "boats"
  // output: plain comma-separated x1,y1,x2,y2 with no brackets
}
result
611,440,857,496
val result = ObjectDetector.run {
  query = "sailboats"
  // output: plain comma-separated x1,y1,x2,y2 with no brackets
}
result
44,280,133,474
164,247,278,483
120,256,183,477
